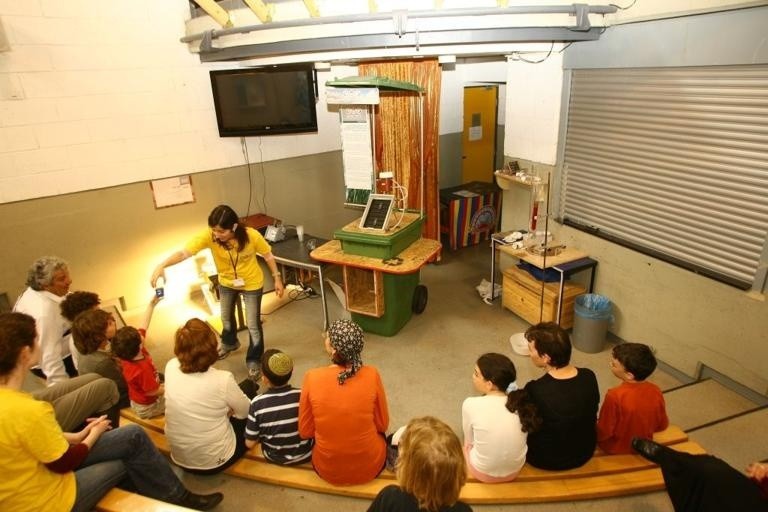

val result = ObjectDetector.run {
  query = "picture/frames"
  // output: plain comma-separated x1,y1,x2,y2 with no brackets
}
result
358,193,397,233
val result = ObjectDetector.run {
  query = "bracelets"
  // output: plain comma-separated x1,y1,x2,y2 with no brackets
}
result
271,271,282,278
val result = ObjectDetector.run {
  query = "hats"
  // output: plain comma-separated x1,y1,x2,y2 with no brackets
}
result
262,349,294,384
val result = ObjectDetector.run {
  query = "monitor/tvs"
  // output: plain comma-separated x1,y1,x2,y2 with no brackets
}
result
209,60,319,139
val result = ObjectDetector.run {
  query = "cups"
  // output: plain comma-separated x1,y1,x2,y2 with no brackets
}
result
155,282,165,300
306,239,316,251
296,224,304,243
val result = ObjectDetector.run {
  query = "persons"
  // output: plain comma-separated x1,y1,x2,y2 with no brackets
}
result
243,348,314,466
593,342,668,456
12,257,164,419
0,313,224,512
630,436,768,512
459,352,527,483
297,318,387,486
149,204,285,380
506,322,600,472
164,319,259,475
366,416,474,512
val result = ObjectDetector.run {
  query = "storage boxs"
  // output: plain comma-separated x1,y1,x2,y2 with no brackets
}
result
332,207,427,260
500,268,588,331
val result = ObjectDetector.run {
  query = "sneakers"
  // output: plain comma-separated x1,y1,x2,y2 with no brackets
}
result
216,340,241,360
246,358,261,382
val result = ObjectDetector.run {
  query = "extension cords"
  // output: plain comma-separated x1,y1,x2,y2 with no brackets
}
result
286,284,312,293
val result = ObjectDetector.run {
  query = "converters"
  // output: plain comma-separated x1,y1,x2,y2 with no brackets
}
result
299,281,307,289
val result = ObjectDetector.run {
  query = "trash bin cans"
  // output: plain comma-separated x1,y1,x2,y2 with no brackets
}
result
572,293,612,353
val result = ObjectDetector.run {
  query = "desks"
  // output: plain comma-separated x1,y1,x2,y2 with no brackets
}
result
254,223,340,333
490,230,599,328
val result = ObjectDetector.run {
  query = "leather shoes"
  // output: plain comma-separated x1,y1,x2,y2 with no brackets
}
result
631,437,679,464
182,492,223,510
